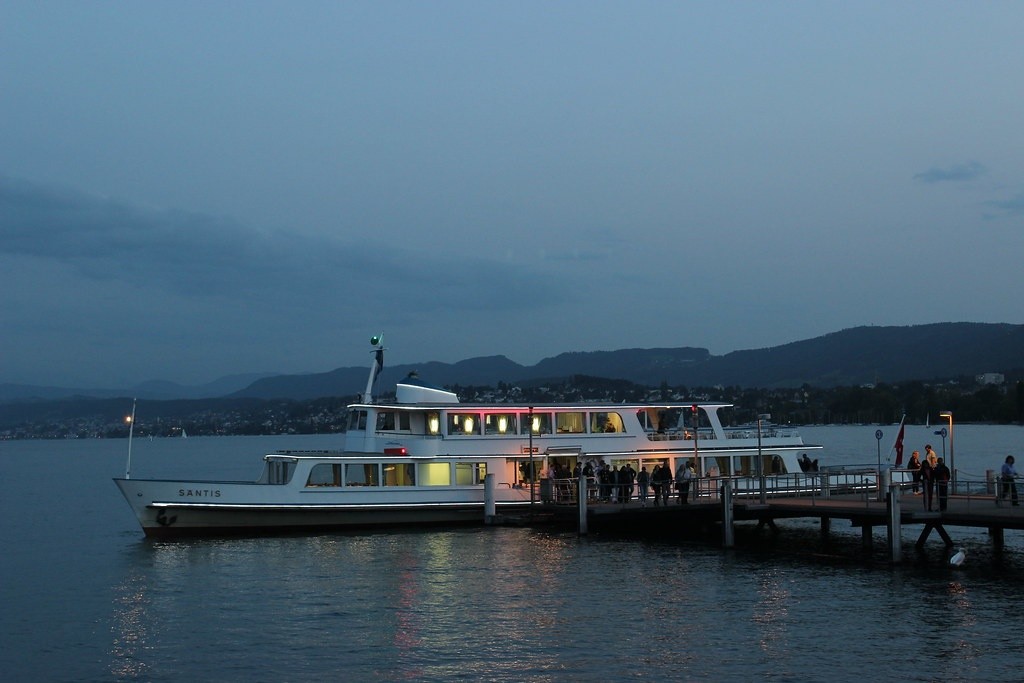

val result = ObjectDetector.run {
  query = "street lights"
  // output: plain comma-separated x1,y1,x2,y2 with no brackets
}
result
690,403,700,500
939,412,955,494
527,405,534,504
757,413,771,504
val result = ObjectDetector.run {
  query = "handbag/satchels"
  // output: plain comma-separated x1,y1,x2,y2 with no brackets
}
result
675,483,679,490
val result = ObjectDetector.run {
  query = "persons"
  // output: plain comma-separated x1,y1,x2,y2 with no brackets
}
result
519,461,531,483
994,455,1021,507
907,451,921,495
772,456,780,473
803,453,819,472
917,444,950,512
711,465,718,477
541,461,699,505
600,417,615,433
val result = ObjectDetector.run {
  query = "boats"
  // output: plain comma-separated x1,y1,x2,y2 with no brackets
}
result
110,331,917,539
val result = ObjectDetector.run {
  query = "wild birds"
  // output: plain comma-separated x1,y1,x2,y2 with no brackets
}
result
950,548,969,571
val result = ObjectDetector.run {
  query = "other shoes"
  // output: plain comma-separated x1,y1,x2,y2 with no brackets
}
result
1012,504,1020,506
913,491,921,495
995,499,999,504
